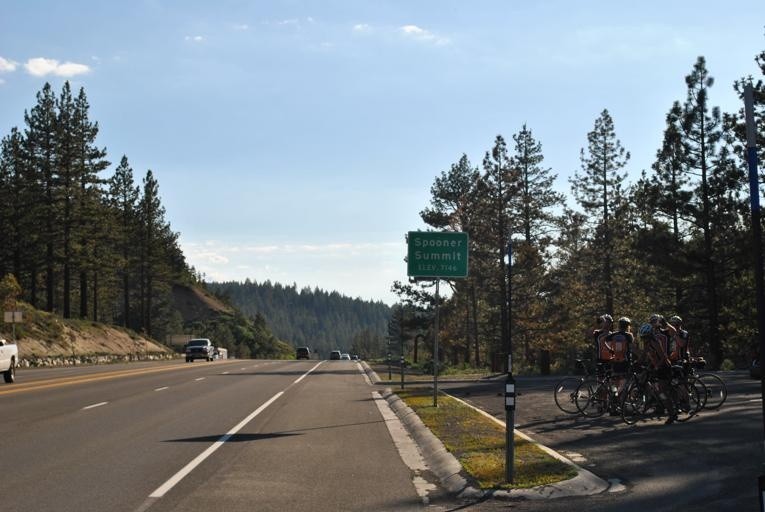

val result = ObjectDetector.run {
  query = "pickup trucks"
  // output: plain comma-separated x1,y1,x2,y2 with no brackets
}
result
0,336,19,383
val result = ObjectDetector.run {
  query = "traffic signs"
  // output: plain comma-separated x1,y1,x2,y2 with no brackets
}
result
407,231,469,278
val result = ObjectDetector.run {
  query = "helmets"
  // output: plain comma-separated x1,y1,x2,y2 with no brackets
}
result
599,314,682,336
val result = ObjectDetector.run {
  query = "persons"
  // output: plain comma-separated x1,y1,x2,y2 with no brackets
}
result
591,311,694,423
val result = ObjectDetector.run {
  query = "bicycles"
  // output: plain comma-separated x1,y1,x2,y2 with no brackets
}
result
554,356,728,425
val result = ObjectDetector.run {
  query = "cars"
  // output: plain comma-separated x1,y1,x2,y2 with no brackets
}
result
330,351,342,360
341,354,350,360
184,338,215,363
350,354,358,360
295,347,311,360
